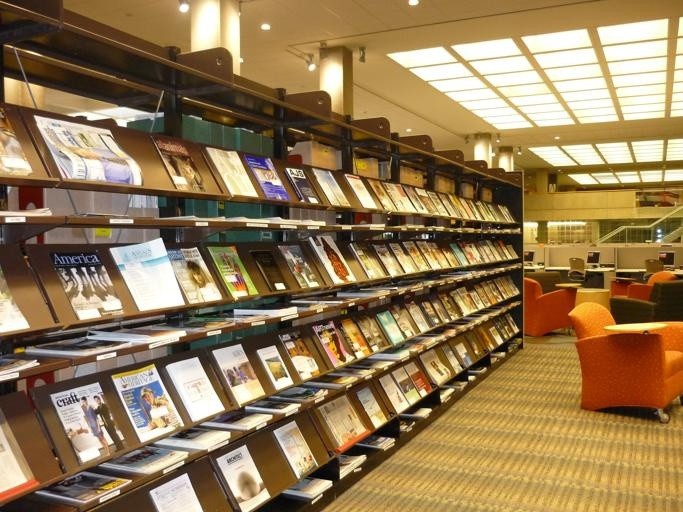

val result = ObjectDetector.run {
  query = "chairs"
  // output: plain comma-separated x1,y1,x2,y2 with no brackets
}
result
524,258,682,422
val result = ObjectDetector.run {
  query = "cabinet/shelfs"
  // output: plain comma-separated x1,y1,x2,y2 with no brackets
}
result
0,0,526,512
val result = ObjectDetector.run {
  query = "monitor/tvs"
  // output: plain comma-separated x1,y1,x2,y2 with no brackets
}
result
524,250,535,265
658,251,676,270
586,251,600,268
